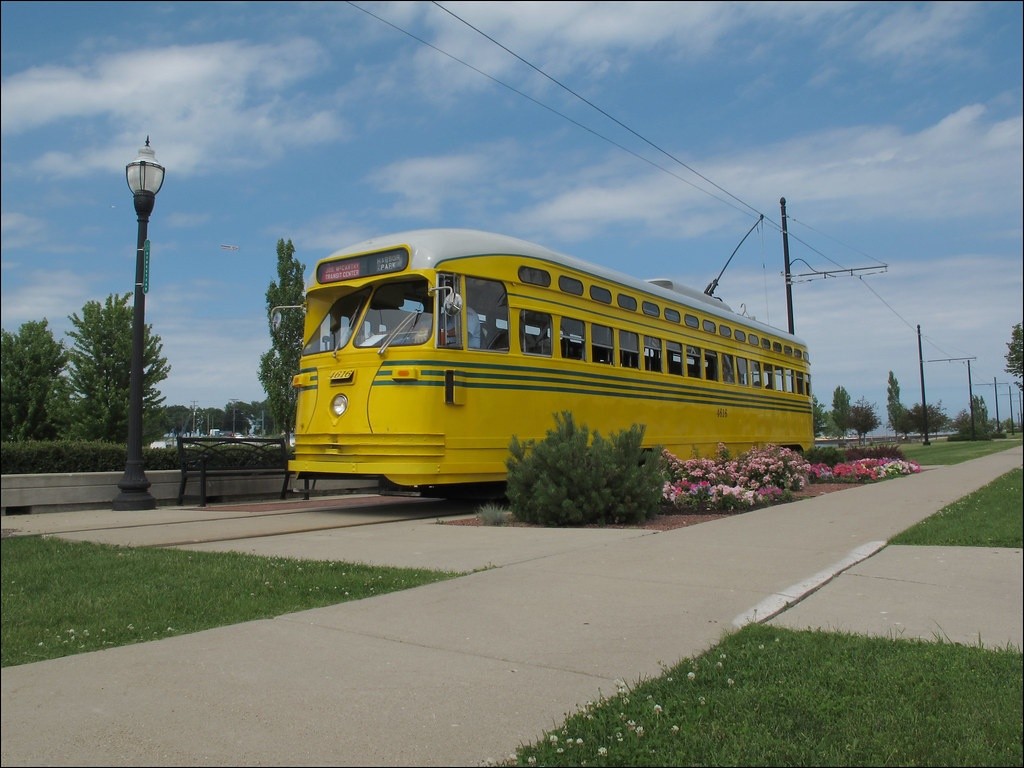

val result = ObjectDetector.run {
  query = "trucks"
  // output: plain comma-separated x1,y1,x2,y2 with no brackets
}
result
210,429,220,437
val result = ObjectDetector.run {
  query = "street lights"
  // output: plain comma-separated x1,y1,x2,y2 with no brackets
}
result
111,136,166,511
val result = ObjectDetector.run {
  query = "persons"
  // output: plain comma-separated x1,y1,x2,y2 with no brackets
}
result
440,302,480,347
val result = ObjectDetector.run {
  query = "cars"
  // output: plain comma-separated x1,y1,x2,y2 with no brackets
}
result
284,433,295,447
219,433,225,436
164,433,175,437
232,432,243,437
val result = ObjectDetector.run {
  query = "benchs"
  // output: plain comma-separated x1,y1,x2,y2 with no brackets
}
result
175,436,308,507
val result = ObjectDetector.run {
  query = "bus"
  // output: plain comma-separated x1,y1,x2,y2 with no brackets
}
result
269,229,815,501
